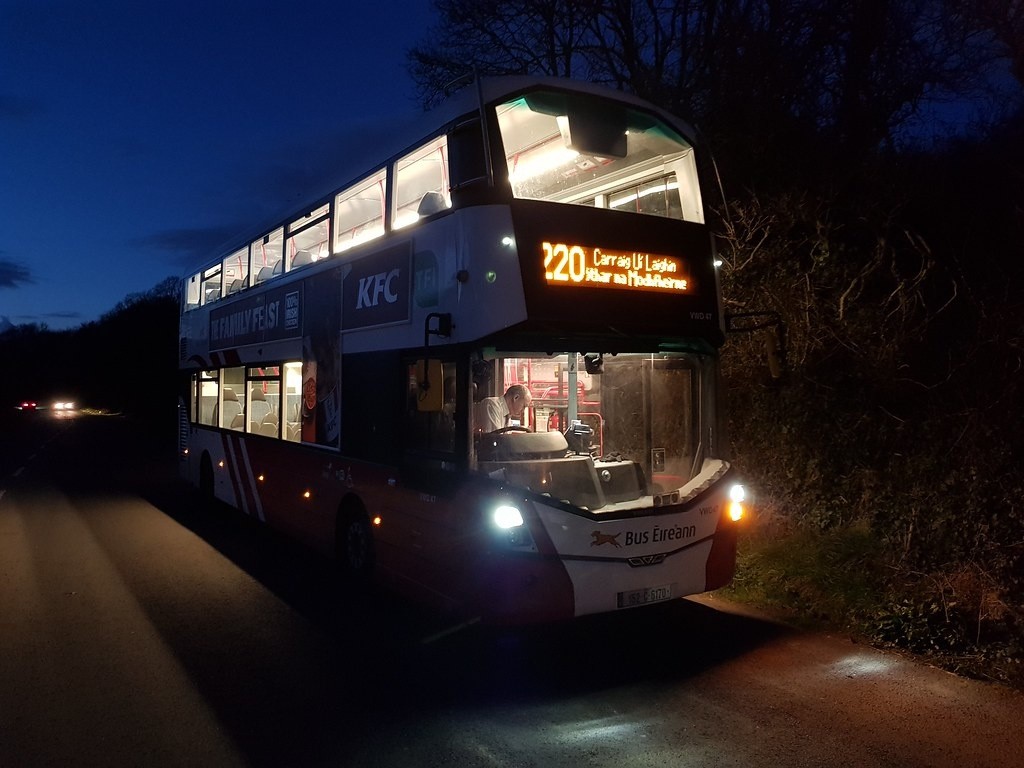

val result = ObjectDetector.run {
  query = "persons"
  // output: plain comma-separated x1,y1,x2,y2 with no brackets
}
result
478,385,532,434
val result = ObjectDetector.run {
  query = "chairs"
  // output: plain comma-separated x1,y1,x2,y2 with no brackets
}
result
251,388,271,423
418,191,448,218
213,388,242,430
208,251,313,305
231,412,301,443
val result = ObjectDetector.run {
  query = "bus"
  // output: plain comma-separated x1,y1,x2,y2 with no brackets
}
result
179,73,787,625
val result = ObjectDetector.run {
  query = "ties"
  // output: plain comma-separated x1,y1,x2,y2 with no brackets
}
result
505,414,509,425
